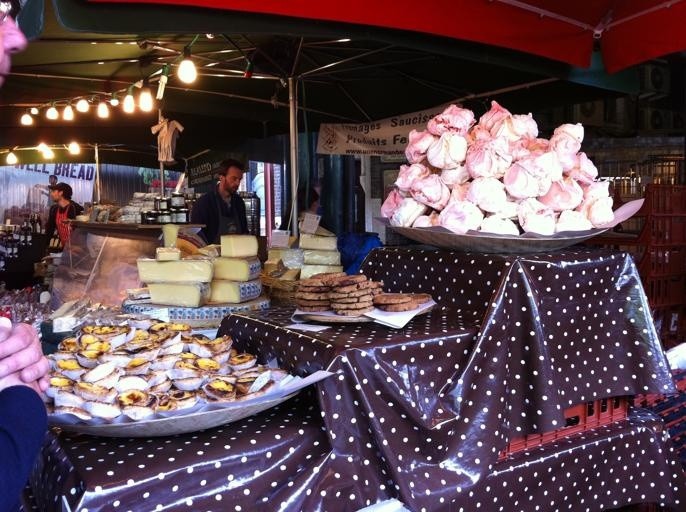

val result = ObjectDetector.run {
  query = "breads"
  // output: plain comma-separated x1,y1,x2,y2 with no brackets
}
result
45,318,286,421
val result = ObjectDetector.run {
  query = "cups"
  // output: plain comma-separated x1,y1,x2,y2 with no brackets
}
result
161,224,179,247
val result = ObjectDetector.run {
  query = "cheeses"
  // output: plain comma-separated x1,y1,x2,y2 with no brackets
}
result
135,223,343,308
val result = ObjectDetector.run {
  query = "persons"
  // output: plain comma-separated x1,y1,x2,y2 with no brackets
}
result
191,158,250,245
0,315,53,512
43,182,84,250
35,175,58,206
278,187,328,233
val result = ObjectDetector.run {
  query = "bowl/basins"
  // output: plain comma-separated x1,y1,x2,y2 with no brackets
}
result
76,216,90,221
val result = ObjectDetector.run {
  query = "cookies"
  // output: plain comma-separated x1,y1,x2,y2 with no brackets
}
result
294,274,433,318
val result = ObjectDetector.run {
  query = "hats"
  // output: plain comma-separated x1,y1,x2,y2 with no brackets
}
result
48,183,72,196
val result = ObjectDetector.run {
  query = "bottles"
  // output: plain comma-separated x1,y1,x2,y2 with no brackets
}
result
141,192,189,224
0,214,41,271
